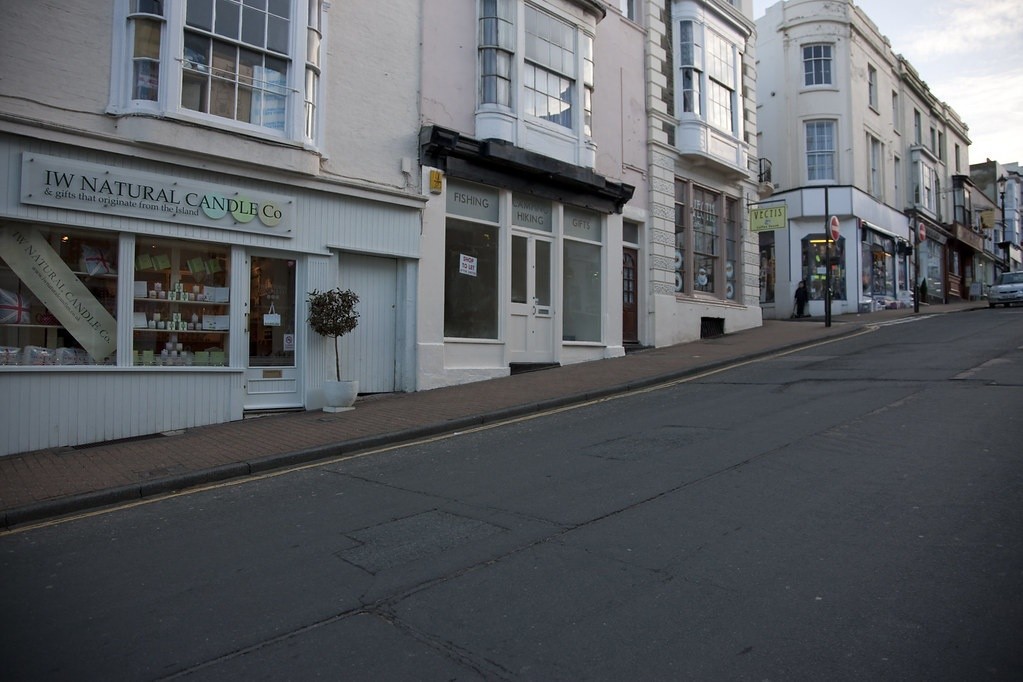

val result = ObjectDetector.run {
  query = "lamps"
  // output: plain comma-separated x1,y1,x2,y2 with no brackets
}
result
970,225,992,241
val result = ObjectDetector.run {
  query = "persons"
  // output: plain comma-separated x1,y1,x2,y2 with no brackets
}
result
794,280,809,318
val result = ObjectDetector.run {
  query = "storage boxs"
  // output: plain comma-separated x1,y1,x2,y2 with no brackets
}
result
135,19,285,130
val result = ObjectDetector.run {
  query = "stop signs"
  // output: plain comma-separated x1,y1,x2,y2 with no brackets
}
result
830,216,840,241
918,223,926,242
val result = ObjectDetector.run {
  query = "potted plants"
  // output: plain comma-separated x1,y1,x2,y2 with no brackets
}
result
306,287,361,413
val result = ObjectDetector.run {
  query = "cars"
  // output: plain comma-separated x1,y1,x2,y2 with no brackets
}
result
987,270,1023,308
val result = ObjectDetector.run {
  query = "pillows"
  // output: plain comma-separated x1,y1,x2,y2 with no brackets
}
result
0,288,33,324
81,244,117,275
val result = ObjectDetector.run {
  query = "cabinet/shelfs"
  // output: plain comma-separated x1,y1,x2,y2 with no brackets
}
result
0,266,117,366
133,268,230,366
